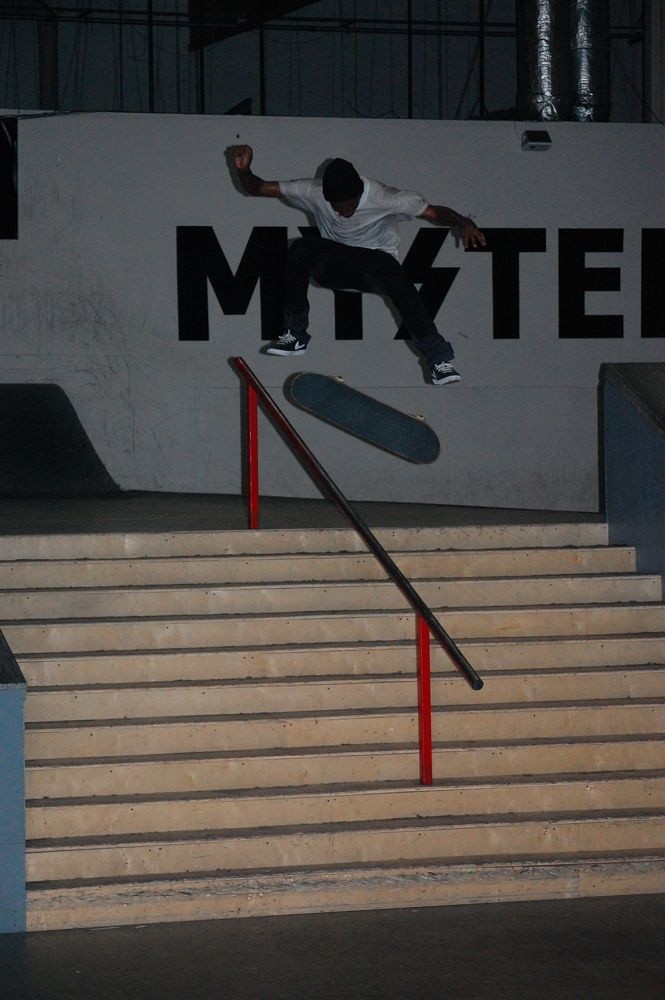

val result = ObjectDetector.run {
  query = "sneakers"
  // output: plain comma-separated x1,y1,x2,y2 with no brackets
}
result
267,330,307,355
431,361,460,384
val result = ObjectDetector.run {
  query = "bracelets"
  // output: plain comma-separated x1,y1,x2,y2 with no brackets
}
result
236,170,253,177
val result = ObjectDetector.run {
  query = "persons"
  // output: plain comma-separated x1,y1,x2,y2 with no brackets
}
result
231,144,485,385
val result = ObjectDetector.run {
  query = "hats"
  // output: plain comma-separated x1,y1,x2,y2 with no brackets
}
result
323,158,364,202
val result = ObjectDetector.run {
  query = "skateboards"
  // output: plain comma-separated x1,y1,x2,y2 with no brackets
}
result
284,370,441,467
